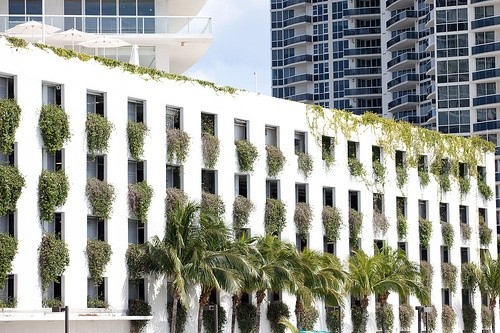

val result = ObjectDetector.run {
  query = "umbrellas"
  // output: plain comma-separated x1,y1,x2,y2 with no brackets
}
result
50,27,96,51
4,19,62,44
77,38,131,58
